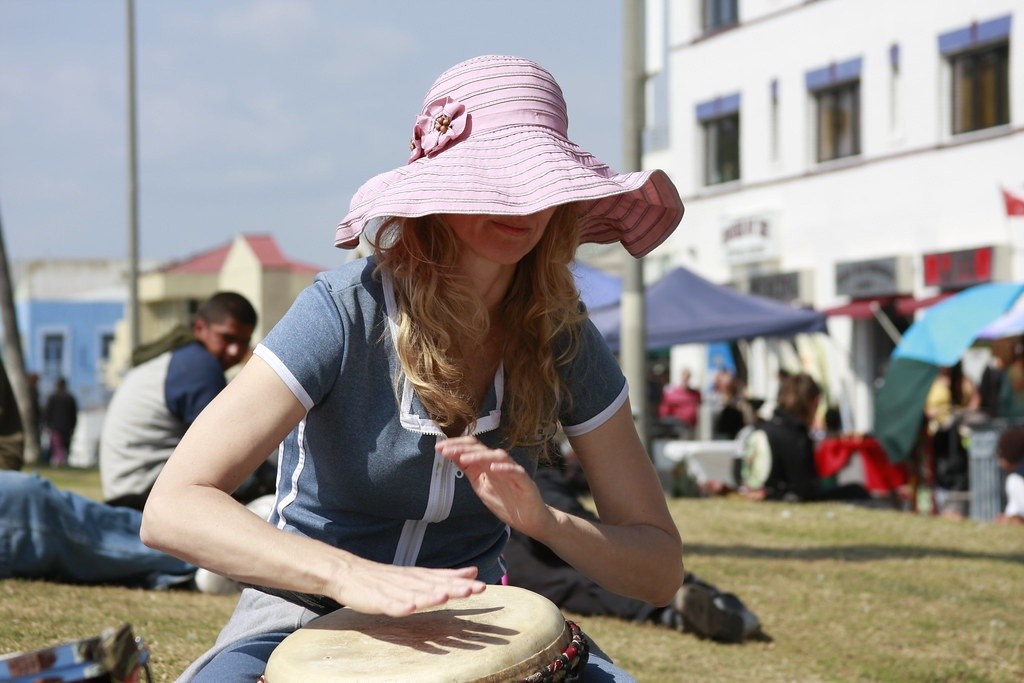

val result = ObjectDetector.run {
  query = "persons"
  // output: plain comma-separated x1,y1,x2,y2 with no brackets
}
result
660,337,1024,519
99,292,279,512
138,54,684,683
44,378,78,468
0,466,277,595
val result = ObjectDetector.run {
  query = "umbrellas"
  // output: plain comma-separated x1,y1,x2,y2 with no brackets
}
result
567,258,1024,464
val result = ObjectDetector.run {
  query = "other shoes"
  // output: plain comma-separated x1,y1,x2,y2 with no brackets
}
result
674,586,745,644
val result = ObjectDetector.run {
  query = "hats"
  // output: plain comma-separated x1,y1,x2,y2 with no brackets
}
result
336,55,684,258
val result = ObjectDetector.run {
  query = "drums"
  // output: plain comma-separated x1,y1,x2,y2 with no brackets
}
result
259,584,590,681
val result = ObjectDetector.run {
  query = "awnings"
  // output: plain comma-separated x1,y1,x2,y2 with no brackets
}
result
896,291,955,315
821,294,888,319
